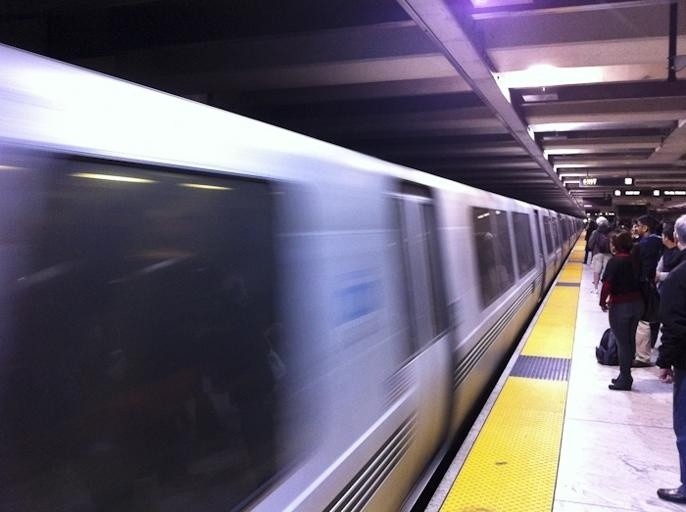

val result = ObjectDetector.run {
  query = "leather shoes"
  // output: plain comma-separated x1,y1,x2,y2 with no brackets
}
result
658,489,686,503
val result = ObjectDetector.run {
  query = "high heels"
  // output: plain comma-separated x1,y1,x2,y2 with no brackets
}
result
609,374,633,390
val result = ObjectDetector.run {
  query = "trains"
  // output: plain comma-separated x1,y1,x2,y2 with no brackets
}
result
0,41,593,511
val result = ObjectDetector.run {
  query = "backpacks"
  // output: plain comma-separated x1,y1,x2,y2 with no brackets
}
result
595,328,618,365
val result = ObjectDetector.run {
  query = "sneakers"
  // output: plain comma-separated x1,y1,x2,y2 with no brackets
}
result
591,289,598,294
632,359,650,367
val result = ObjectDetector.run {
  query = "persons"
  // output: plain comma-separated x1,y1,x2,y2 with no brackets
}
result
583,214,686,504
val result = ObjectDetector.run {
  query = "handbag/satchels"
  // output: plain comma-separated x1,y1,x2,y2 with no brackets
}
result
643,282,660,322
